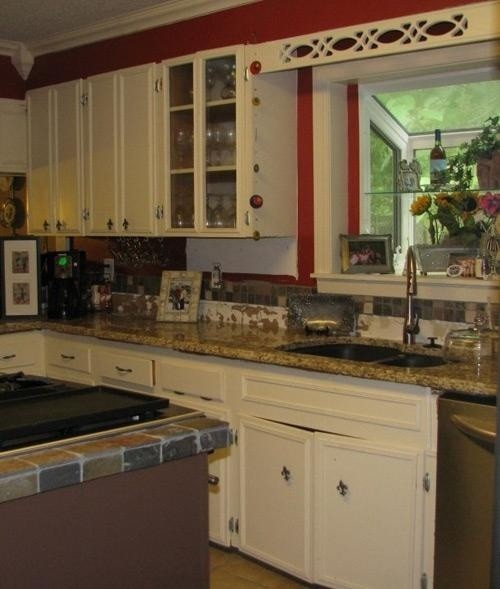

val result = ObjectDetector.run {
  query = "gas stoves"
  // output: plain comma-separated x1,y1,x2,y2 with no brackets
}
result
0,371,171,452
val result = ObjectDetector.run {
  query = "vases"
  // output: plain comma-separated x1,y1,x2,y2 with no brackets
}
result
413,243,496,275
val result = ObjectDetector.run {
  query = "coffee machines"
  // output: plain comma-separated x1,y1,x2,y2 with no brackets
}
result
40,248,88,322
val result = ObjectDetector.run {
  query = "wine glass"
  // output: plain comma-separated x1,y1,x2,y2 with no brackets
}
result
201,62,238,100
175,124,240,168
173,191,238,229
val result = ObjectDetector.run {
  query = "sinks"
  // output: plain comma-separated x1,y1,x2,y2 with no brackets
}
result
275,333,406,364
374,347,460,370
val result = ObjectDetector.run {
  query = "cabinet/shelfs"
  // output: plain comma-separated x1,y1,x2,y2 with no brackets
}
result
83,61,159,236
26,76,84,235
235,356,434,589
161,43,301,240
45,329,93,388
155,349,235,546
1,97,26,175
98,340,154,396
0,332,41,375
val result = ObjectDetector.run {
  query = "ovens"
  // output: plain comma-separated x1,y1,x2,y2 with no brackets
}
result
435,392,499,588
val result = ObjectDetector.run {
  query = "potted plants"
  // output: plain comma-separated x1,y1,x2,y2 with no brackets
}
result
448,116,500,188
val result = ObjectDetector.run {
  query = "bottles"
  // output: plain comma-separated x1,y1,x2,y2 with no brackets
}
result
91,276,113,311
429,128,448,184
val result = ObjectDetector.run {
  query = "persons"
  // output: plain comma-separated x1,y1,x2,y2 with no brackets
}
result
174,285,187,309
349,243,375,264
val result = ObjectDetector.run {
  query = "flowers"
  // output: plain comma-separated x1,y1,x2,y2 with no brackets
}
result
408,190,498,243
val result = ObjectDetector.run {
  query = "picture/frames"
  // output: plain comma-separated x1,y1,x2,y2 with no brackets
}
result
155,269,205,325
2,240,40,316
337,232,397,277
394,159,423,193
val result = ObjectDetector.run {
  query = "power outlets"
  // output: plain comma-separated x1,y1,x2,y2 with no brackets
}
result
103,258,115,273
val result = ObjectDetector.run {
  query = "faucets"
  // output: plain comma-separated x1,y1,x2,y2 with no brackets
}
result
399,245,420,345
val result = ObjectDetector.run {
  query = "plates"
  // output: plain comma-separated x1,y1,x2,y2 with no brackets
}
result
0,175,16,191
0,198,20,229
